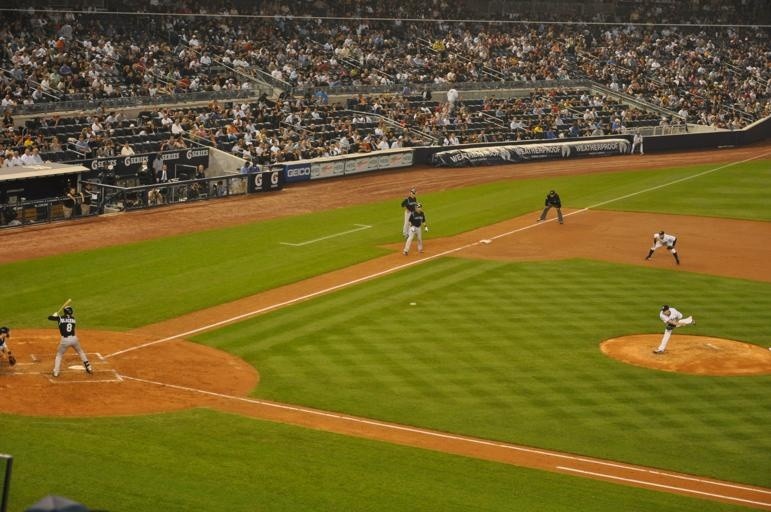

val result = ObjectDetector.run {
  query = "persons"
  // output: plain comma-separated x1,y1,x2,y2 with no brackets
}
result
402,203,429,256
536,189,564,225
388,0,771,156
401,188,417,238
0,1,401,230
653,304,695,353
0,325,12,360
48,305,93,377
645,230,680,265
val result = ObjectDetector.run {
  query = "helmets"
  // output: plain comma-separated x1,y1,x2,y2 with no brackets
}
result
64,306,73,315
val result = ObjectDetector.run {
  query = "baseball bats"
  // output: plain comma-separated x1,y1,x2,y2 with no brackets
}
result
57,299,71,315
409,302,415,306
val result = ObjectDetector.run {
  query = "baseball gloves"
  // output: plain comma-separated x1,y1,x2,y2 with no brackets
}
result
666,324,675,329
9,356,15,365
